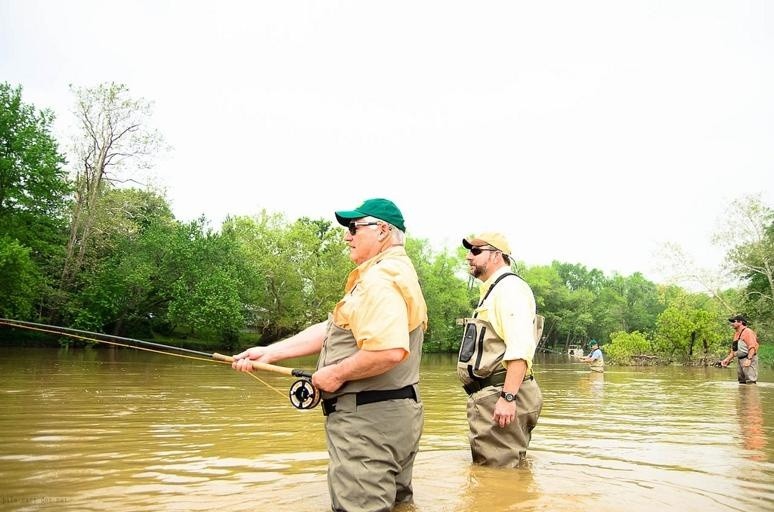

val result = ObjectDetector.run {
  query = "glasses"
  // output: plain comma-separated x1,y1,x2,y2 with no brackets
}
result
348,222,391,235
470,247,496,256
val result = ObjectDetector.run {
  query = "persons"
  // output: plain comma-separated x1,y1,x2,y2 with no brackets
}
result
456,230,546,471
721,315,759,385
230,197,429,512
578,338,603,372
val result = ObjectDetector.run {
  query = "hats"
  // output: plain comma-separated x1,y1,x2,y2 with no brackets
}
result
729,315,746,323
334,199,405,233
462,232,511,257
586,339,596,346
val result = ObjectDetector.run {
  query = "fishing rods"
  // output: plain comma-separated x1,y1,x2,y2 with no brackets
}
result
0,317,321,408
536,346,586,361
666,360,722,368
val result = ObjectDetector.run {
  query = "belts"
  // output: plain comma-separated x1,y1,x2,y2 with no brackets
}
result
739,353,757,359
462,371,506,395
321,384,415,416
